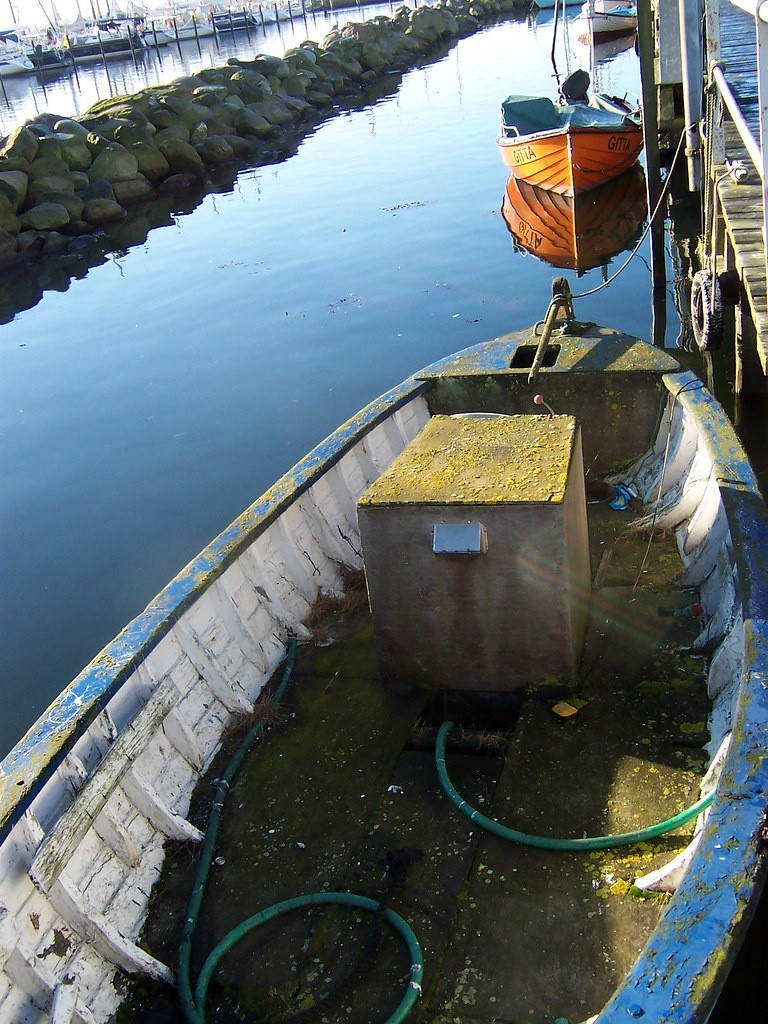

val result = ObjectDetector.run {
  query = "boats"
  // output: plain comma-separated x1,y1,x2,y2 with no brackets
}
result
0,275,768,1023
571,26,638,71
500,162,646,288
0,0,307,76
497,0,644,199
569,0,638,33
